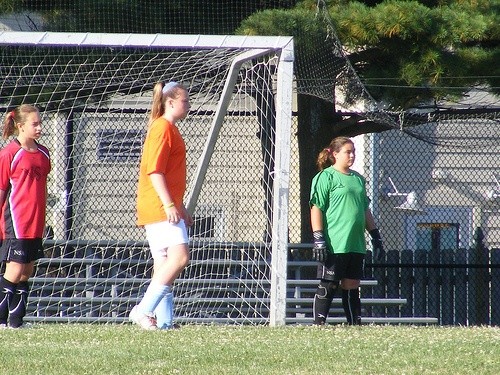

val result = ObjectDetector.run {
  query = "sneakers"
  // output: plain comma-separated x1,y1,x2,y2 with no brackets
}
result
129,305,157,329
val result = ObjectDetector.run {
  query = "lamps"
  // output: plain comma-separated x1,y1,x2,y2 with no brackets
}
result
388,176,424,212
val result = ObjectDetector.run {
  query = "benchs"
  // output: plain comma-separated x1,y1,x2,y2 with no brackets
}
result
23,240,439,323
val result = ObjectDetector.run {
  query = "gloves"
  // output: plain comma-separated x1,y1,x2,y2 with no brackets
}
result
312,231,331,262
369,228,385,261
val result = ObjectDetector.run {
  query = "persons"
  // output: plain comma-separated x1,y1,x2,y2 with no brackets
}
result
0,105,51,328
127,82,195,334
309,137,383,327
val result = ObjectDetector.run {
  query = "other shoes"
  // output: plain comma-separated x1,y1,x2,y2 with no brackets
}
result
11,323,31,329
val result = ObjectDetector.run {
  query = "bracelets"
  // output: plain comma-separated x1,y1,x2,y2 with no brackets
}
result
164,203,174,209
